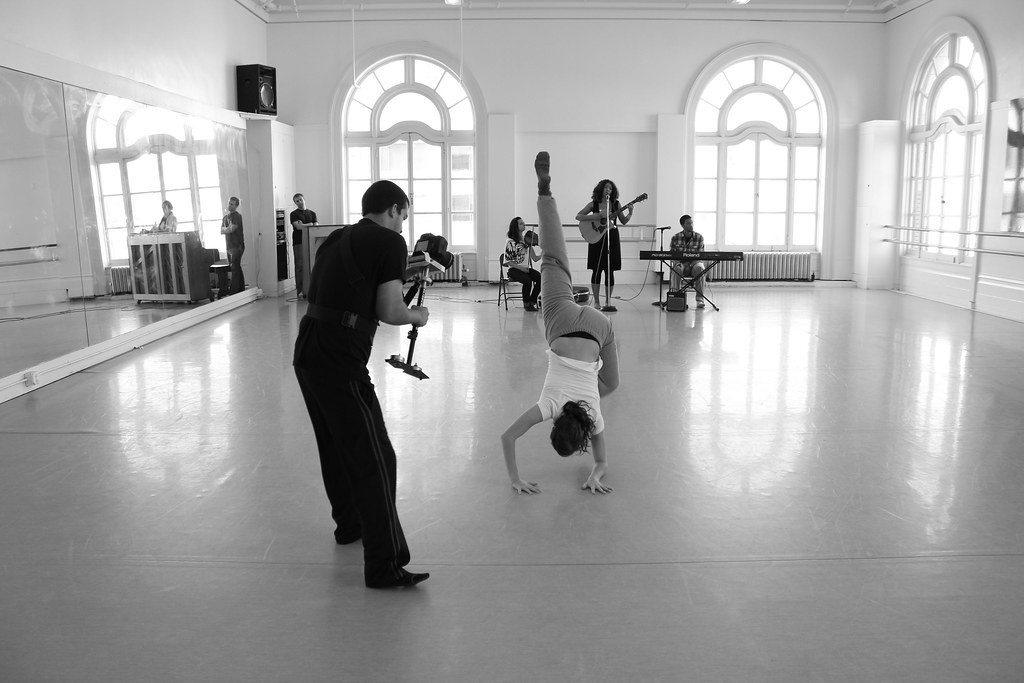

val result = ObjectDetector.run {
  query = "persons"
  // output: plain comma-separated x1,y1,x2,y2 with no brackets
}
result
669,215,705,307
157,200,177,232
293,180,430,588
221,197,245,295
501,151,620,495
290,193,319,298
504,217,542,311
576,179,634,309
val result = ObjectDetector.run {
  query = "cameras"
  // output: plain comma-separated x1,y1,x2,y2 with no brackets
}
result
413,233,454,270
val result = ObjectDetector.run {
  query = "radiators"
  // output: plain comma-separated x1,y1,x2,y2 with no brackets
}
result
706,252,811,282
111,266,132,295
407,253,462,282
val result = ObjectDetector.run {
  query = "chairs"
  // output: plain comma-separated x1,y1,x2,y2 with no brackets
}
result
498,253,539,310
669,260,704,291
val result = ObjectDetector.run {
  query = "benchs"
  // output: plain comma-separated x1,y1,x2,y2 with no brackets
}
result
209,264,231,299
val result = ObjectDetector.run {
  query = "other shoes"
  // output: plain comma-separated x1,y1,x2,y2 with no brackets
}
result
524,303,538,311
697,301,706,309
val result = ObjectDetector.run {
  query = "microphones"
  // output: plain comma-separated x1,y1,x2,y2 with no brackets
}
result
656,226,672,230
606,194,610,199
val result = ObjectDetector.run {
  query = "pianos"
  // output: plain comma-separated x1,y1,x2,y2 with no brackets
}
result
639,249,745,312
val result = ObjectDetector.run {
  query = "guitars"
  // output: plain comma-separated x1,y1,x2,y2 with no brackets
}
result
579,192,649,245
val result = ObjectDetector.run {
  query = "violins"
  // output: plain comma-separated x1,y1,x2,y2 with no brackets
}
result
524,230,538,246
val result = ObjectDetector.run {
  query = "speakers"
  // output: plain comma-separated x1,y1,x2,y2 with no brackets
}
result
667,292,687,312
237,64,277,116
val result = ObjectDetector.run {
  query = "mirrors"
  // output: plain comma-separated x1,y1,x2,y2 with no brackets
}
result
0,37,258,380
